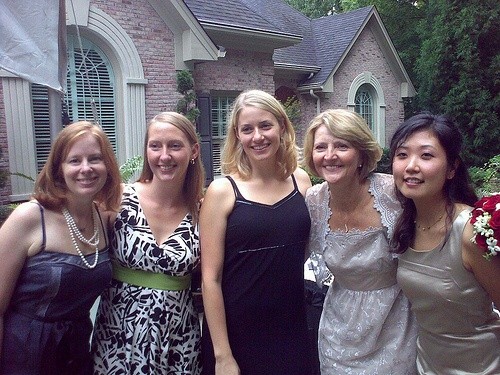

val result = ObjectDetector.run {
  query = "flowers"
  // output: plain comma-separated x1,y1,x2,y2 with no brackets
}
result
469,192,500,263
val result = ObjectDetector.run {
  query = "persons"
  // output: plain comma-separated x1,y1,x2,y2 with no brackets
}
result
197,91,316,375
386,115,500,375
90,112,210,375
303,109,419,375
0,121,122,375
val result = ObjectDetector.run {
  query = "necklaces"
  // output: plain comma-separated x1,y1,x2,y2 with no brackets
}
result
414,208,447,231
57,199,100,269
